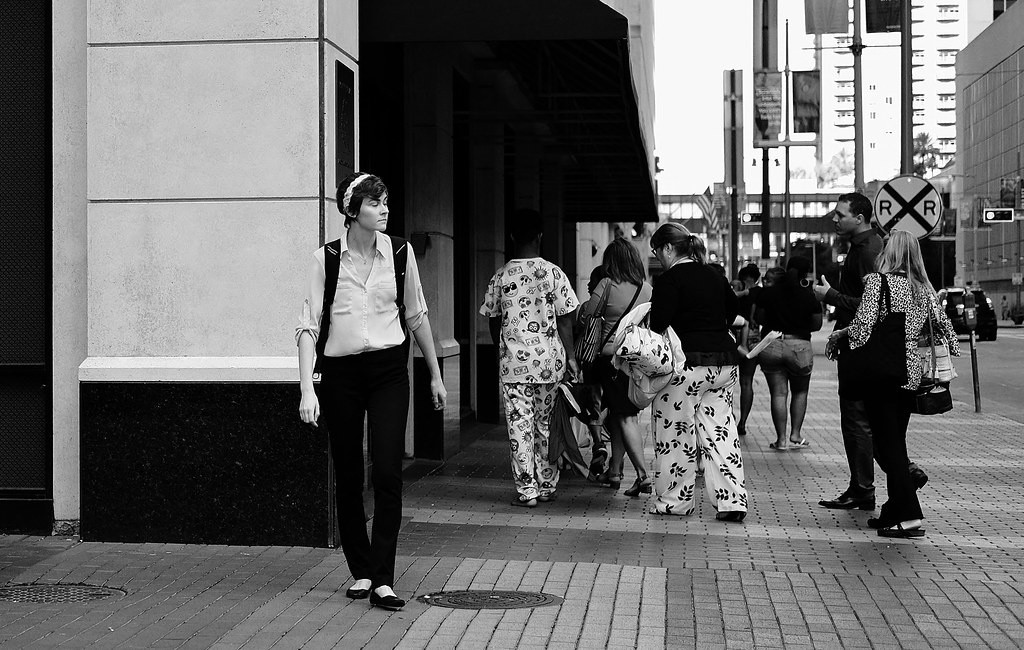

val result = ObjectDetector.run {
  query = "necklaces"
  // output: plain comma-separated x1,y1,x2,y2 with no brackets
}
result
347,240,372,264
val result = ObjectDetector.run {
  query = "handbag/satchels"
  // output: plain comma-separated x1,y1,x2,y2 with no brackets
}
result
910,378,954,415
846,272,909,389
616,310,673,379
574,277,612,369
923,344,959,384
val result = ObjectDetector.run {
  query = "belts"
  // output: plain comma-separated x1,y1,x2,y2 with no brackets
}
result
776,334,804,339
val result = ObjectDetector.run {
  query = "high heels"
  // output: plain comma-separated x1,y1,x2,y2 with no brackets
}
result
624,475,652,497
593,469,621,490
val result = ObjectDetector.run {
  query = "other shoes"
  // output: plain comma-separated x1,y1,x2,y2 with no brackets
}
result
537,496,550,502
737,426,747,436
370,589,405,608
511,498,537,507
609,456,624,479
715,511,747,522
649,506,686,517
346,580,373,599
589,442,608,476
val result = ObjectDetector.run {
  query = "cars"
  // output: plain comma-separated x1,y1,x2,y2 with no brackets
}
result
936,287,998,342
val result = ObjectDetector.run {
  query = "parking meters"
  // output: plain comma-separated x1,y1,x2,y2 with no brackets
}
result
961,286,983,412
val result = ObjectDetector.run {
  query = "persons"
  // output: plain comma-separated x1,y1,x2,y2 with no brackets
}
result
552,238,654,508
813,193,928,510
642,223,752,521
479,207,580,507
1000,295,1010,320
295,172,447,609
708,256,823,451
828,229,960,537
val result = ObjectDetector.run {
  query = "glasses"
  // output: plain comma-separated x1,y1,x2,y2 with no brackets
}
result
651,241,675,256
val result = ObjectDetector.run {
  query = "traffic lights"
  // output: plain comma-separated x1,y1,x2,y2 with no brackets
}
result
740,212,762,225
983,208,1014,223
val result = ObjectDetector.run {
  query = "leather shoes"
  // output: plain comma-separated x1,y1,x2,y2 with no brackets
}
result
818,490,875,511
908,462,928,492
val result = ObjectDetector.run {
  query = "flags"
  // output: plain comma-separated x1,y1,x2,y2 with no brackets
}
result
696,187,720,235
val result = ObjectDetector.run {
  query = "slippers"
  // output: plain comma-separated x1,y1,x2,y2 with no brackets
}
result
770,442,787,450
868,518,895,529
877,522,925,537
789,438,810,450
